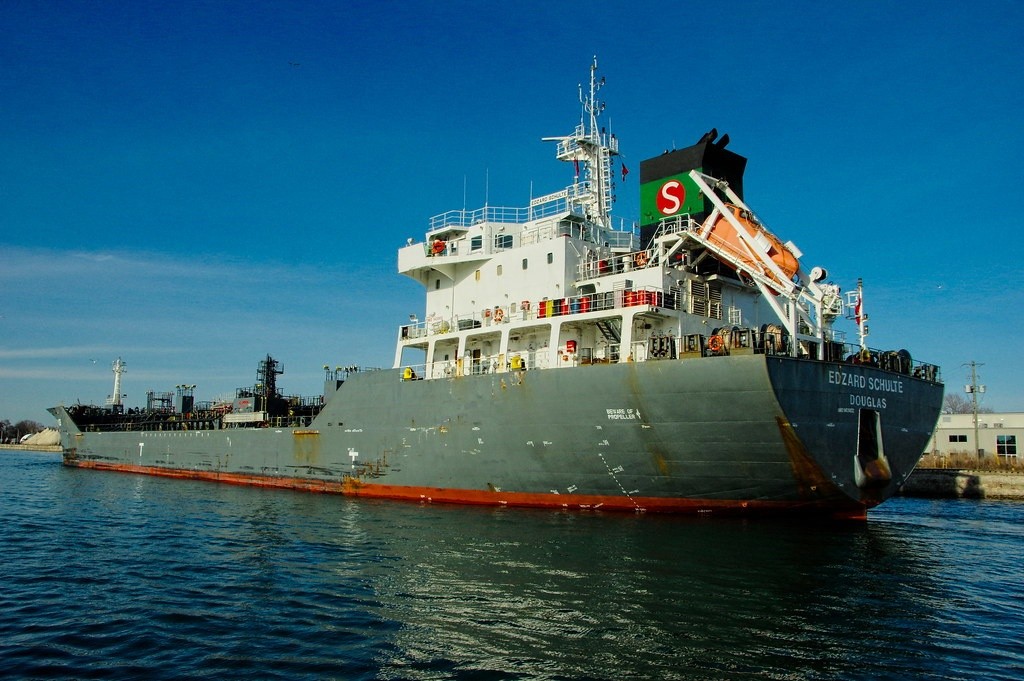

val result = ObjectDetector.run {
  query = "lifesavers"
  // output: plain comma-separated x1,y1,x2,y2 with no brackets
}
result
709,335,724,351
494,309,503,321
636,253,647,265
433,241,445,252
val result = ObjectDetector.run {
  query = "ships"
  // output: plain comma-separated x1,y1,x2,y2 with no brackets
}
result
46,44,945,525
